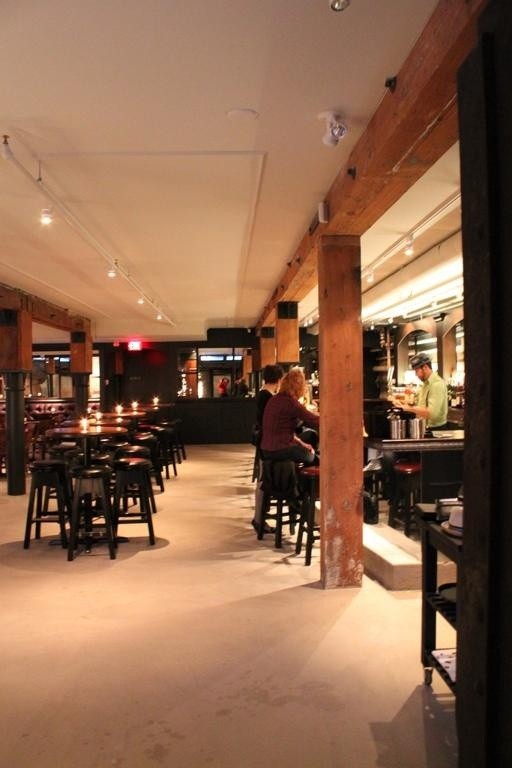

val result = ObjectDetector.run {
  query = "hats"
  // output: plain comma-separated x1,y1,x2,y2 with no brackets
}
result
409,353,433,370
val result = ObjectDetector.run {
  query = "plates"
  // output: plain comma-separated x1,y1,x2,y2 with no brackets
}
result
442,521,463,536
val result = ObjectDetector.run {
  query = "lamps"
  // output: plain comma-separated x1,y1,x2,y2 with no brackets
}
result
314,106,349,149
362,230,418,283
38,198,57,226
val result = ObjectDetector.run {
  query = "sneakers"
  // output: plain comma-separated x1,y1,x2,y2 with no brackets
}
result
251,519,276,534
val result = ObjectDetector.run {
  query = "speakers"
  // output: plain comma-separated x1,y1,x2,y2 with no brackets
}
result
317,200,330,224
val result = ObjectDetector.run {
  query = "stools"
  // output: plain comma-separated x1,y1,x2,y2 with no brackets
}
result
0,407,187,560
258,456,425,567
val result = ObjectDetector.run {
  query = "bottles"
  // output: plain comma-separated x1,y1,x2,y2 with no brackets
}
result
397,381,420,405
448,506,463,530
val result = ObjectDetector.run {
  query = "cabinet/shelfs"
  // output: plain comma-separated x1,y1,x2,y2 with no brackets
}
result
406,505,463,700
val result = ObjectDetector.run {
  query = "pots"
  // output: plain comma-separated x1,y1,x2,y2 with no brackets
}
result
384,407,423,439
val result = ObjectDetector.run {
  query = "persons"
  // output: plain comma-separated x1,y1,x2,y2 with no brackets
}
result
217,378,229,397
232,377,248,397
401,351,447,438
251,366,283,533
261,367,319,466
